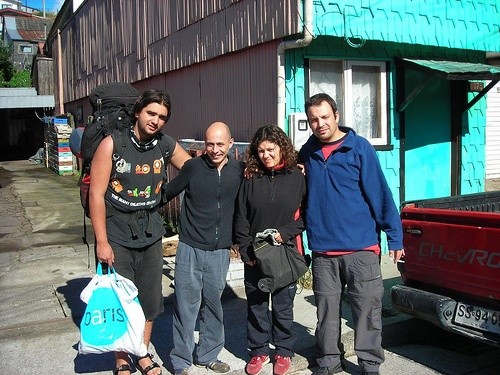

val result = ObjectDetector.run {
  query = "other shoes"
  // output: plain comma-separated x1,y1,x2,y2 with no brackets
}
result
360,371,380,375
311,361,345,375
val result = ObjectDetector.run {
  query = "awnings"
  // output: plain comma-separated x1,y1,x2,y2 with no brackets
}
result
402,54,500,80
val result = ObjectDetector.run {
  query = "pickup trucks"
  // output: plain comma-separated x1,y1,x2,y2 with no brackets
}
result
391,190,500,351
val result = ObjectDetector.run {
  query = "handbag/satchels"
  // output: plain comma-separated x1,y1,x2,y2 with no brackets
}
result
78,262,148,357
253,229,311,293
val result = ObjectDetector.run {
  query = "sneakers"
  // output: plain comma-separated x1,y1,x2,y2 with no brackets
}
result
194,358,230,374
273,354,290,375
246,355,270,375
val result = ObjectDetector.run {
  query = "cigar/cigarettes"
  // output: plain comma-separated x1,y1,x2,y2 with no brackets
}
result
391,259,405,264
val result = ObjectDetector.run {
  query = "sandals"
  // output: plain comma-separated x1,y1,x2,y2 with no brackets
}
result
135,353,162,375
113,364,133,375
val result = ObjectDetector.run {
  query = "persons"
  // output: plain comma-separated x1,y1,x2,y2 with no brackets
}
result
159,120,305,375
244,93,405,375
89,90,192,375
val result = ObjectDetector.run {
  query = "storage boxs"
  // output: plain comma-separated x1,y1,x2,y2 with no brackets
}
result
43,116,73,176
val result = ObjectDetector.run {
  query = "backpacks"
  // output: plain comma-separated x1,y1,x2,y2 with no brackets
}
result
80,83,169,237
